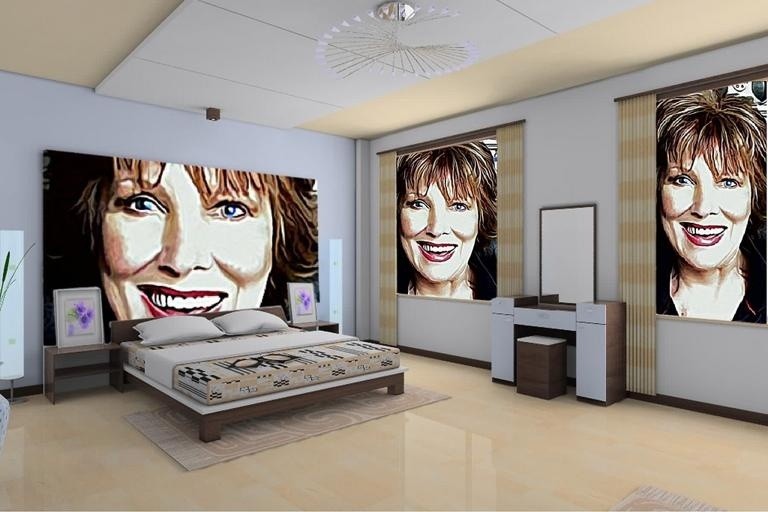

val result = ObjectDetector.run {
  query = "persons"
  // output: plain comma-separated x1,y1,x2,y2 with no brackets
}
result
397,140,496,300
655,89,765,322
75,155,317,325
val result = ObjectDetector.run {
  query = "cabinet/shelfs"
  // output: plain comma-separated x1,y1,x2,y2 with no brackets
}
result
541,294,559,303
491,294,627,406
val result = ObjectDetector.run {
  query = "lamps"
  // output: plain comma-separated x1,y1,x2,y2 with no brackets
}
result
313,1,479,80
328,239,346,334
0,229,31,404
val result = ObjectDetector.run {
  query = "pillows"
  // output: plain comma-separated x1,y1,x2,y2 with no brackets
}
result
133,315,225,347
210,309,292,336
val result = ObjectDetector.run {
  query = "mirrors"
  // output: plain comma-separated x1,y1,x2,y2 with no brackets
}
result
538,205,598,304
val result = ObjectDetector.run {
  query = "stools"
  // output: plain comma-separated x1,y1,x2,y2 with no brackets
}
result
516,334,569,400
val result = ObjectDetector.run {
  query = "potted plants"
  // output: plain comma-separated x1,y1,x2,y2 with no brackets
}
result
0,241,37,447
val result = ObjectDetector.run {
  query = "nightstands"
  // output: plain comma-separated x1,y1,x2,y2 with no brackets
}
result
42,343,124,403
289,320,341,335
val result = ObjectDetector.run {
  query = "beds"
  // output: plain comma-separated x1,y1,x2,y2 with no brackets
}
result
108,306,407,443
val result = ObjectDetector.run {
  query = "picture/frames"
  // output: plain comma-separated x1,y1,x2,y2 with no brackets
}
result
53,287,105,347
286,282,318,323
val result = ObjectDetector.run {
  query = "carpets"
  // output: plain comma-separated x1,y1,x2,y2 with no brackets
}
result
120,380,452,474
607,484,730,511
